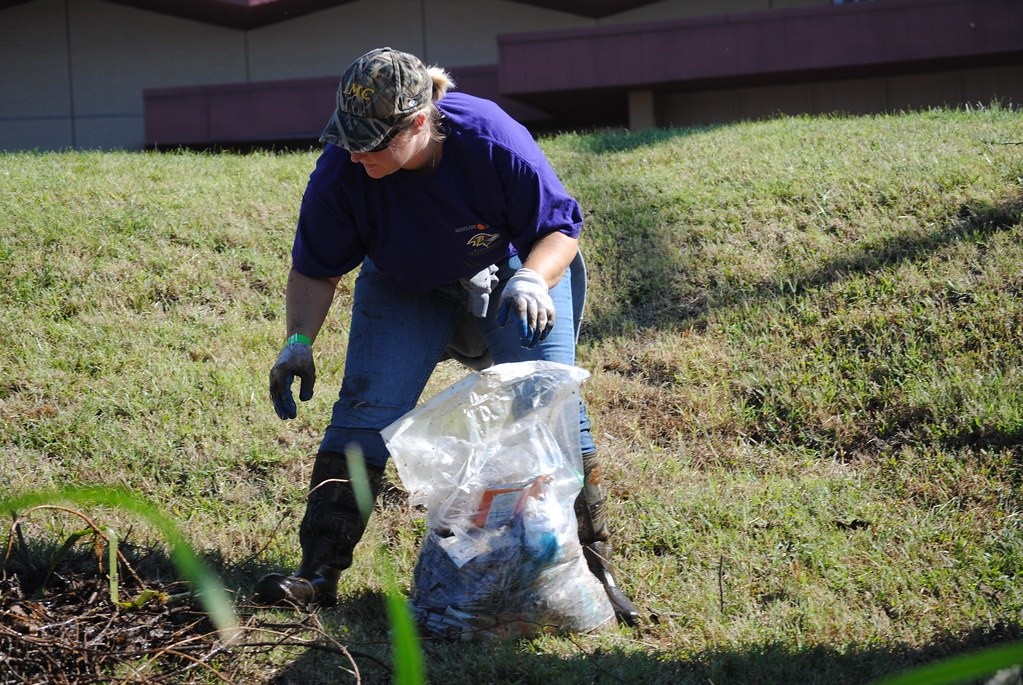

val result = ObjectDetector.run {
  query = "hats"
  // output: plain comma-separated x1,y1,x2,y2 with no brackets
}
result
319,46,434,154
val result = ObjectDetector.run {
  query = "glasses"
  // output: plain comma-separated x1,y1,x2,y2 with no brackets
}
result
369,126,402,154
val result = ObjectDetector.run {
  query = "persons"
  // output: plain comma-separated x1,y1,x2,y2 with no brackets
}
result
254,47,640,627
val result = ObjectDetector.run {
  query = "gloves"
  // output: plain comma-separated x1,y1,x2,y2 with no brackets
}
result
269,334,315,420
496,268,556,351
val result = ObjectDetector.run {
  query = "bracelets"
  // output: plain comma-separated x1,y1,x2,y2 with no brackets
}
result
287,332,313,346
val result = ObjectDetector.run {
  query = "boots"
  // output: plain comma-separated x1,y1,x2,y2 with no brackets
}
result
575,452,642,626
253,450,382,609
